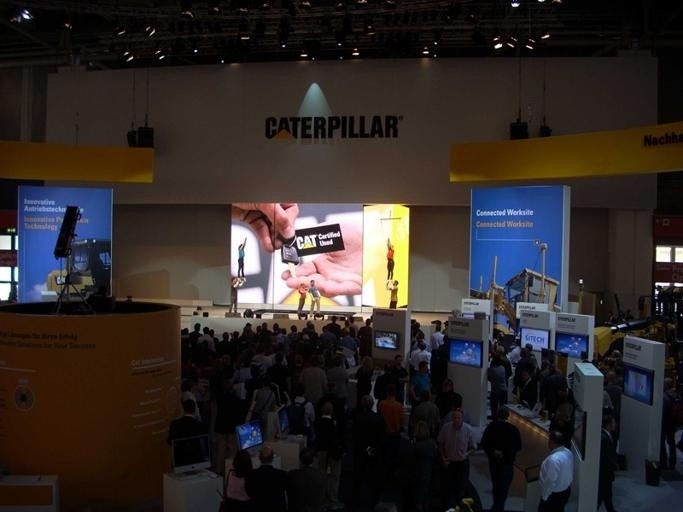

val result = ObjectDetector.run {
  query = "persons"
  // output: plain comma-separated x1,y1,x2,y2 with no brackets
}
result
298,282,308,315
386,237,395,280
229,277,246,313
238,238,247,278
231,203,300,253
281,223,362,298
308,280,321,314
170,317,683,512
387,280,398,308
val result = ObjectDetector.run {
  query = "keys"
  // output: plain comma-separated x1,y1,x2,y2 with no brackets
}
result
281,235,299,279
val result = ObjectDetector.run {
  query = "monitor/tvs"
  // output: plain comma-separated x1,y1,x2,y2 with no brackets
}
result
374,331,398,350
171,434,211,477
449,338,483,368
235,420,264,452
554,331,588,361
620,363,654,405
520,326,551,353
571,404,587,460
276,410,291,436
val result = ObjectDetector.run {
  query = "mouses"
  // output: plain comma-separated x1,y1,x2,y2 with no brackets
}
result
296,434,304,438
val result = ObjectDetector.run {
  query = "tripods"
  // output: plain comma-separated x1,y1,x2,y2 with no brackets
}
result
53,258,95,312
